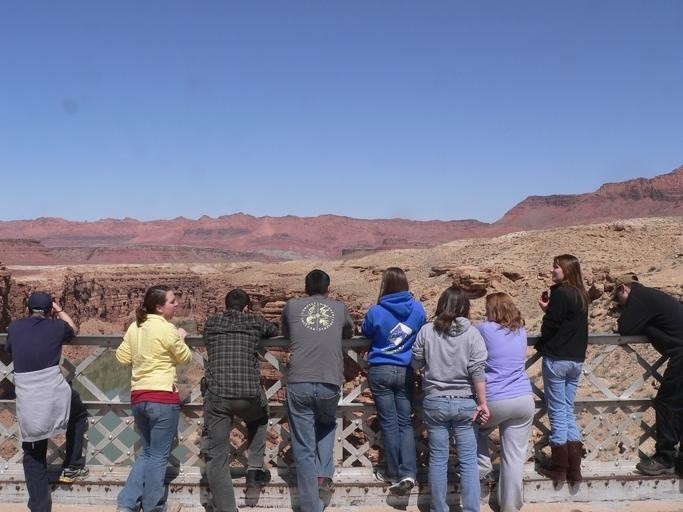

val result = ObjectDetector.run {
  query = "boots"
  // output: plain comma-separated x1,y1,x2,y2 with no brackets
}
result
568,440,584,481
538,444,568,481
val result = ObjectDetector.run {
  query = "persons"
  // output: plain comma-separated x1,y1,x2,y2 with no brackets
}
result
477,293,534,510
282,269,353,511
363,267,427,495
202,287,279,511
614,272,682,477
115,284,191,512
411,288,491,512
4,292,90,512
533,255,587,482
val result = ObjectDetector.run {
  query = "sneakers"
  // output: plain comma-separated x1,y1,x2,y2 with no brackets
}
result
318,476,334,492
59,469,88,483
636,458,675,475
163,498,181,511
247,469,271,486
375,467,415,496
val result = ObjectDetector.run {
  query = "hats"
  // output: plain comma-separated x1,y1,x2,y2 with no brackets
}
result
609,272,638,297
29,291,53,310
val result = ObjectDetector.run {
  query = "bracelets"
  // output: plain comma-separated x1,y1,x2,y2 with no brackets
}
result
56,307,64,313
